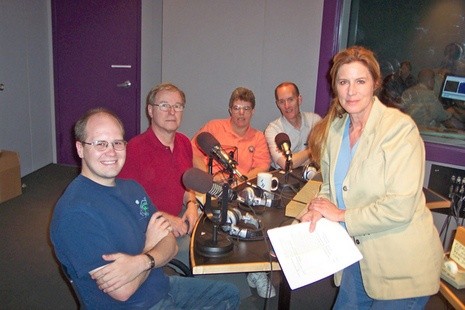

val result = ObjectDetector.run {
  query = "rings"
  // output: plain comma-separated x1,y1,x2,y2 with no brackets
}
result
168,226,173,232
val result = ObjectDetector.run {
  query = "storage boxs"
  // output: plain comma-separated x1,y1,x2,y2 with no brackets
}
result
0,150,22,203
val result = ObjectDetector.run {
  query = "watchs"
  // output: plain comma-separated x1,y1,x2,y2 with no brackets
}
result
186,200,200,208
143,253,155,271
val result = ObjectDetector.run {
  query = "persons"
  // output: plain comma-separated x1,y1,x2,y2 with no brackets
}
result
299,46,443,310
191,87,271,188
49,110,240,310
117,85,200,274
380,62,453,128
265,82,323,170
433,41,465,96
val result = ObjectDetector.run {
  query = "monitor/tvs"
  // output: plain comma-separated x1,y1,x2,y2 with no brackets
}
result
439,72,465,107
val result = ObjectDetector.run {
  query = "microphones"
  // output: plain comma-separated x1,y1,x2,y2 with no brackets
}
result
196,132,249,184
275,133,293,167
182,168,246,205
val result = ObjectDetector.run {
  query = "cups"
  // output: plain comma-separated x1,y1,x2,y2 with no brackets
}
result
257,172,279,192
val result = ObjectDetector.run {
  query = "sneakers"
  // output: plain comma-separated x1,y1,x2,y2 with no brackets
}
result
247,272,275,298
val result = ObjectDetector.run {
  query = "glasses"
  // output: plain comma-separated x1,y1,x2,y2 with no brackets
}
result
231,104,252,114
149,102,185,111
79,139,128,152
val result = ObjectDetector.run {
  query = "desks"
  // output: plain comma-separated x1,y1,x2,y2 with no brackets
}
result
190,168,452,310
